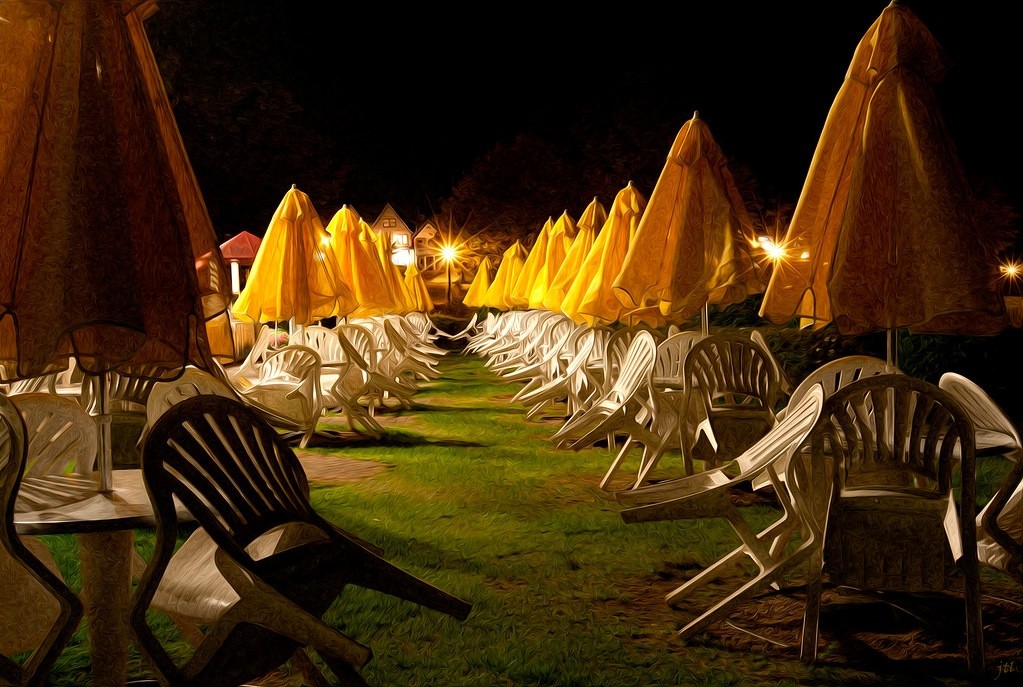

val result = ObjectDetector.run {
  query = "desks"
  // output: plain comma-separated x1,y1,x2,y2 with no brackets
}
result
319,360,348,375
13,468,239,686
801,425,1017,460
650,375,706,390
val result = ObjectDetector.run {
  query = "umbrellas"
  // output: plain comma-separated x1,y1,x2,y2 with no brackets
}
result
461,0,1014,437
0,0,437,490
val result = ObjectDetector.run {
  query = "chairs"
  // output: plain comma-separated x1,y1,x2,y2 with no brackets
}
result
0,308,1023,687
